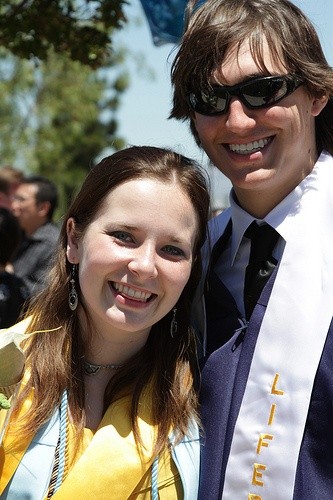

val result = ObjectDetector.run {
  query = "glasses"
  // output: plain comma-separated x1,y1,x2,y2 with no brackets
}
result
9,194,39,203
189,73,306,117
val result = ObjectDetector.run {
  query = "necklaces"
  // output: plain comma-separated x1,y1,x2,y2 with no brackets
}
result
77,356,125,377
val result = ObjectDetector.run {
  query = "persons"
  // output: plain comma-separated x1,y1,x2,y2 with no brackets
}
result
166,0,333,500
0,165,62,330
0,145,210,500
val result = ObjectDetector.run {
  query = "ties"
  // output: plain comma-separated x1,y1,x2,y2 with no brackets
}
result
244,221,286,323
